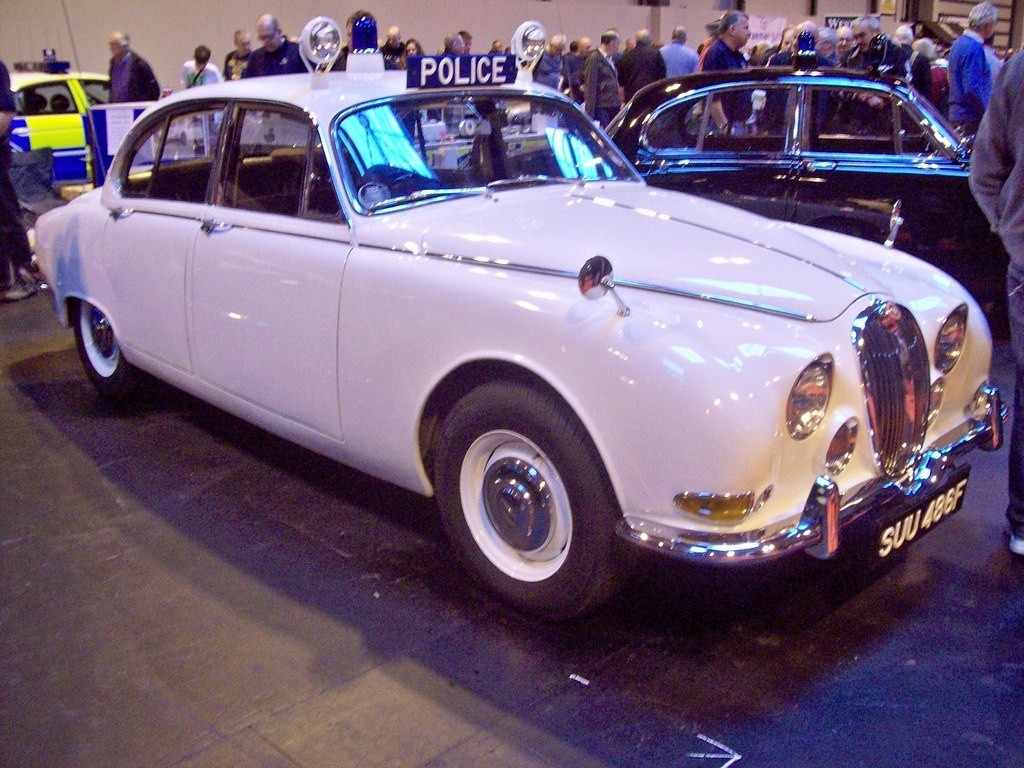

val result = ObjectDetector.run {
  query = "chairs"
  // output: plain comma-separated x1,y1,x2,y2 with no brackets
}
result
27,94,46,114
50,94,70,112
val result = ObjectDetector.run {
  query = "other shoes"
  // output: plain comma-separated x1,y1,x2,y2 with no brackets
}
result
1004,515,1024,554
0,272,41,300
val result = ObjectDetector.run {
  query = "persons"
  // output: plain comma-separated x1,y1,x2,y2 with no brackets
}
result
982,31,1016,83
108,30,160,102
478,10,949,134
439,30,472,55
223,28,253,81
329,11,398,72
380,26,404,71
181,44,223,90
397,38,425,71
968,47,1024,554
0,61,38,299
247,13,314,75
947,2,999,134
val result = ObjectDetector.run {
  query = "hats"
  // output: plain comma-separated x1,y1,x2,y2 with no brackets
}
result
707,19,721,33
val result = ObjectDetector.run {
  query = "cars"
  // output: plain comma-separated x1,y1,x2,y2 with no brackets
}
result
592,69,1009,329
0,67,162,184
32,69,1007,614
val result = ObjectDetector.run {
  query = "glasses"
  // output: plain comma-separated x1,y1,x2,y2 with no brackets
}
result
258,29,277,41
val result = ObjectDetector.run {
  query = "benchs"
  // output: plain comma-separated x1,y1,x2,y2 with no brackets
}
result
129,149,325,214
